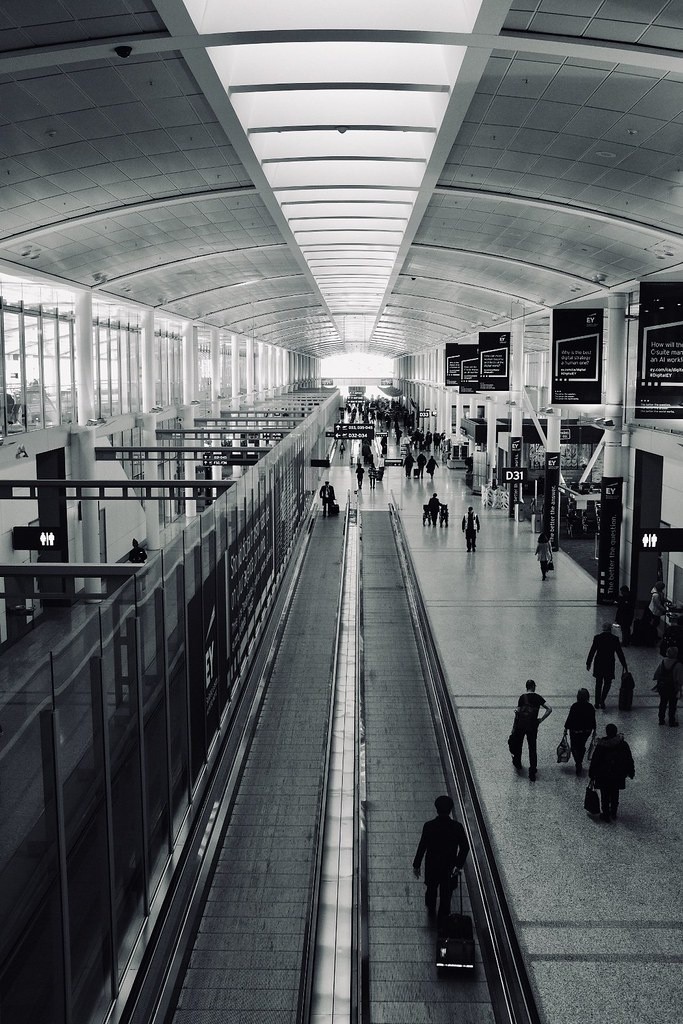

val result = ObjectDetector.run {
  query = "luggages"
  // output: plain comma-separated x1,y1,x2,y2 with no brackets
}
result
619,667,633,711
436,871,476,970
414,462,420,476
330,499,339,516
377,468,384,481
611,624,622,643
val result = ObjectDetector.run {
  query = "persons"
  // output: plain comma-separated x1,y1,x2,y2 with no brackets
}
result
462,506,481,554
345,394,451,462
586,622,629,708
128,536,148,562
338,441,345,458
428,493,445,528
354,453,385,489
414,795,470,925
510,679,552,778
465,452,473,474
586,723,635,818
535,533,554,581
402,452,439,480
7,392,13,420
319,481,336,521
616,580,670,647
653,646,682,724
563,688,595,777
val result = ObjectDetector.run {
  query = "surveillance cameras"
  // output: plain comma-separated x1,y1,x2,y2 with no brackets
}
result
412,278,416,280
115,46,133,58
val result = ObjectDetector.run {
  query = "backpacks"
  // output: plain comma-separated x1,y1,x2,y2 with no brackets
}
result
652,660,679,698
340,445,344,450
660,625,683,662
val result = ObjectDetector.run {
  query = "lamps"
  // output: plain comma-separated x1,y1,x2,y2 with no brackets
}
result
0,395,225,448
402,379,615,427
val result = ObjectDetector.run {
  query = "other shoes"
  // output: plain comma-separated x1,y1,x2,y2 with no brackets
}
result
670,722,679,727
600,702,605,709
609,813,617,819
594,704,599,709
659,720,665,725
600,813,610,822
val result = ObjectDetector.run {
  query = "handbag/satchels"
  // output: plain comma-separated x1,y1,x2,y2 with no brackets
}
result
427,469,430,472
583,779,600,814
514,694,532,733
547,560,554,570
508,736,521,756
556,735,571,762
587,732,595,759
622,671,635,689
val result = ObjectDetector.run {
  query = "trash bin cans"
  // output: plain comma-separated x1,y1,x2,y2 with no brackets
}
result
531,513,540,533
514,501,524,522
595,533,600,559
